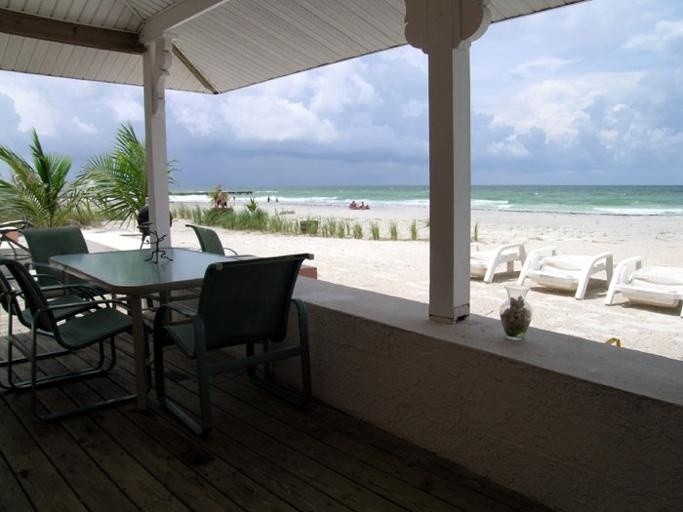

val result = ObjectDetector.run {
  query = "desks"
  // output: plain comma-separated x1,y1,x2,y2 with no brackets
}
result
47,245,248,415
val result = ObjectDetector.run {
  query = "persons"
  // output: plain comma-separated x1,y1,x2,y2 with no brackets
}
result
348,200,356,209
358,201,369,209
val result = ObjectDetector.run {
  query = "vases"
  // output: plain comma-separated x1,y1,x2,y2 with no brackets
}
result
499,283,532,340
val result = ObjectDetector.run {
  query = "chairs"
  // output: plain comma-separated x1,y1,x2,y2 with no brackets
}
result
517,246,617,301
20,225,121,330
471,241,528,284
0,254,155,422
156,246,319,439
0,267,112,388
604,257,683,319
1,221,43,266
187,224,257,258
